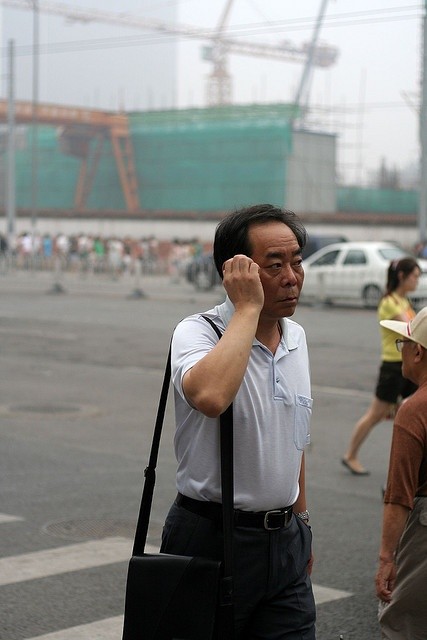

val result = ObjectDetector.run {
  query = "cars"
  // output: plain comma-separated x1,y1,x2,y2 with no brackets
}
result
297,242,426,309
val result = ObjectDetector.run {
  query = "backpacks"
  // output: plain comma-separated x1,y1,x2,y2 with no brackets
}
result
122,315,234,640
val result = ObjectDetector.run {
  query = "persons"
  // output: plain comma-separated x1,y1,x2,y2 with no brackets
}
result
340,257,419,478
159,205,320,634
0,232,203,288
378,302,427,634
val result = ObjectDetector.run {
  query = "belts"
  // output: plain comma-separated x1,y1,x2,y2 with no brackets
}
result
175,492,294,530
